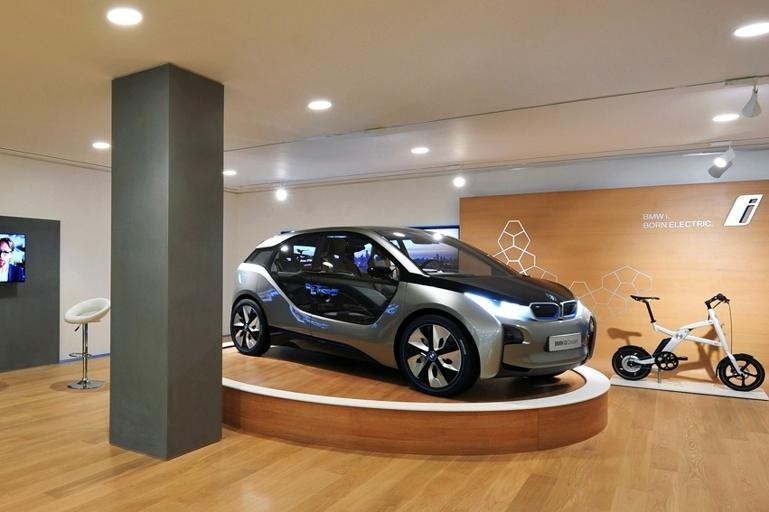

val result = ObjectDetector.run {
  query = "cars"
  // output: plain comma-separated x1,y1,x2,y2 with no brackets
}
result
230,222,597,394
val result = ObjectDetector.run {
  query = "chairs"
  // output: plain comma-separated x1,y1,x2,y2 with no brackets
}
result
273,250,391,281
64,298,110,389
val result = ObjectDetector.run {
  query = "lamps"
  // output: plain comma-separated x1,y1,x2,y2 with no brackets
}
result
741,83,762,118
713,144,734,168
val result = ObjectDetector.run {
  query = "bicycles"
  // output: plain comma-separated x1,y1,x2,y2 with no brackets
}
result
612,291,765,394
